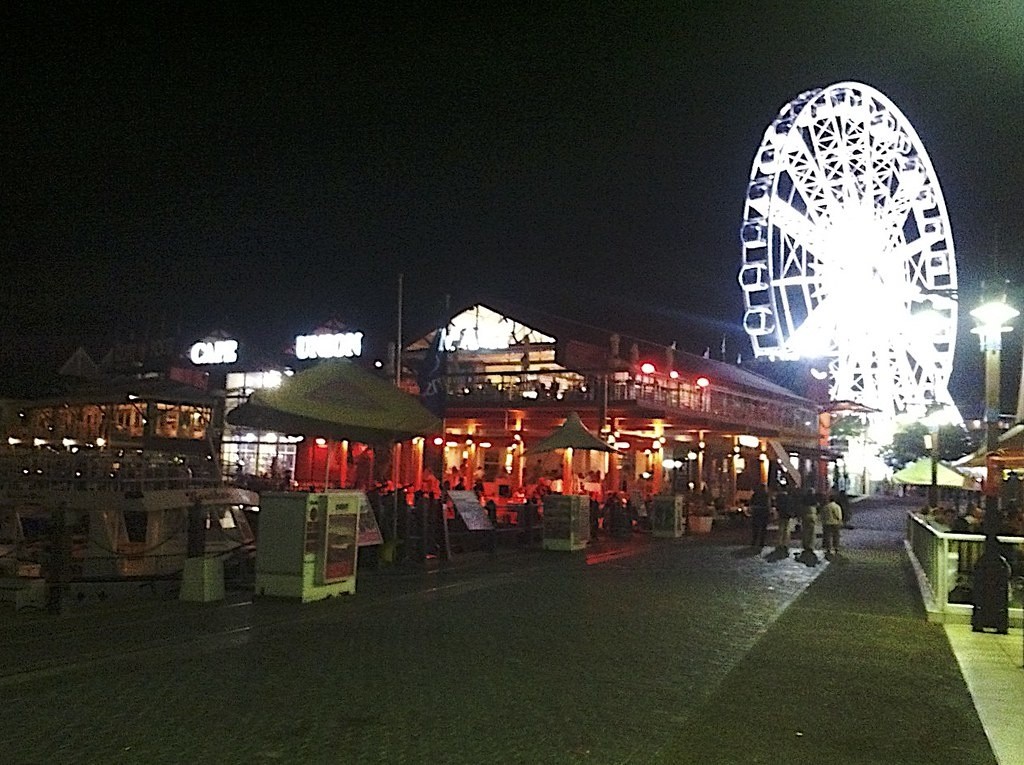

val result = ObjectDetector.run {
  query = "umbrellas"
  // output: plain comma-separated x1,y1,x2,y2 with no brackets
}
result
225,356,442,493
517,411,627,495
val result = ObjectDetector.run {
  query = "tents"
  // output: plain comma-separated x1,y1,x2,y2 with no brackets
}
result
893,424,1024,490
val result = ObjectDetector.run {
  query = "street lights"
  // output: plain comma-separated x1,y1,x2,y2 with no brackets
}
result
968,279,1021,451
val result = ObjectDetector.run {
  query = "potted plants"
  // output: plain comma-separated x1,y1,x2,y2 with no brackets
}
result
688,502,714,533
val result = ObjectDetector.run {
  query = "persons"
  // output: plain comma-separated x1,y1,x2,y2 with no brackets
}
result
749,483,842,551
921,472,1024,537
439,458,685,530
